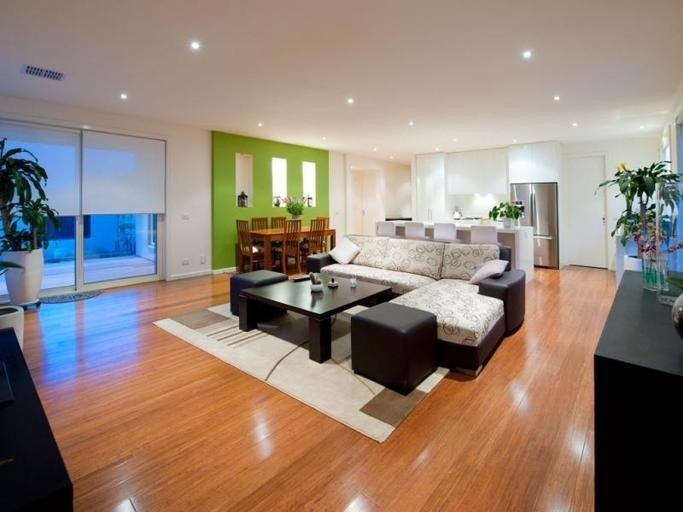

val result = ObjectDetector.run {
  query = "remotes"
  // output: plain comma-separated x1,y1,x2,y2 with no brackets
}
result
293,276,310,282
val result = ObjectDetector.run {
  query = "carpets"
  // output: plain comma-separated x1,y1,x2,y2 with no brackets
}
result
151,301,450,442
37,289,103,304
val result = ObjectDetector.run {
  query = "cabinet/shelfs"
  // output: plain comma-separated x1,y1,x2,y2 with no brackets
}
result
593,268,683,512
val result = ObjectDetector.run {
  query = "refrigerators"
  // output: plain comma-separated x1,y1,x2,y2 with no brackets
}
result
509,182,560,269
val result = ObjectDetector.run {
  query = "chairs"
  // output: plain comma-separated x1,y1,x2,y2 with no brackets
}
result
376,221,402,238
404,221,431,240
235,216,336,276
470,225,504,245
433,223,463,243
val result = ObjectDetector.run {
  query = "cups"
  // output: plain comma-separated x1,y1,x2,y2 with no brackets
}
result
350,273,357,288
274,196,281,207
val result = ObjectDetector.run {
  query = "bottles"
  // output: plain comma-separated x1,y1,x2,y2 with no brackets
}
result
238,191,248,207
308,195,312,207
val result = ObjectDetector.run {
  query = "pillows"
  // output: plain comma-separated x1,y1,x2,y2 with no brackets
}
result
471,258,509,285
329,237,360,264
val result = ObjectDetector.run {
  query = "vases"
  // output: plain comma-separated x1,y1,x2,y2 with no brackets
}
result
639,240,670,292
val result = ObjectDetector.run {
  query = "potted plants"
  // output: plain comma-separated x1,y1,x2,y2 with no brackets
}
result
488,201,525,228
0,139,49,354
592,161,683,285
284,195,306,226
0,198,63,306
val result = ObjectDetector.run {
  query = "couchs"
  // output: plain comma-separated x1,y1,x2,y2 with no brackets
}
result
306,234,525,379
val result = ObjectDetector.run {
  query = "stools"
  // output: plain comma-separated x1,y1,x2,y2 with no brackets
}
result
230,269,288,320
351,301,438,396
